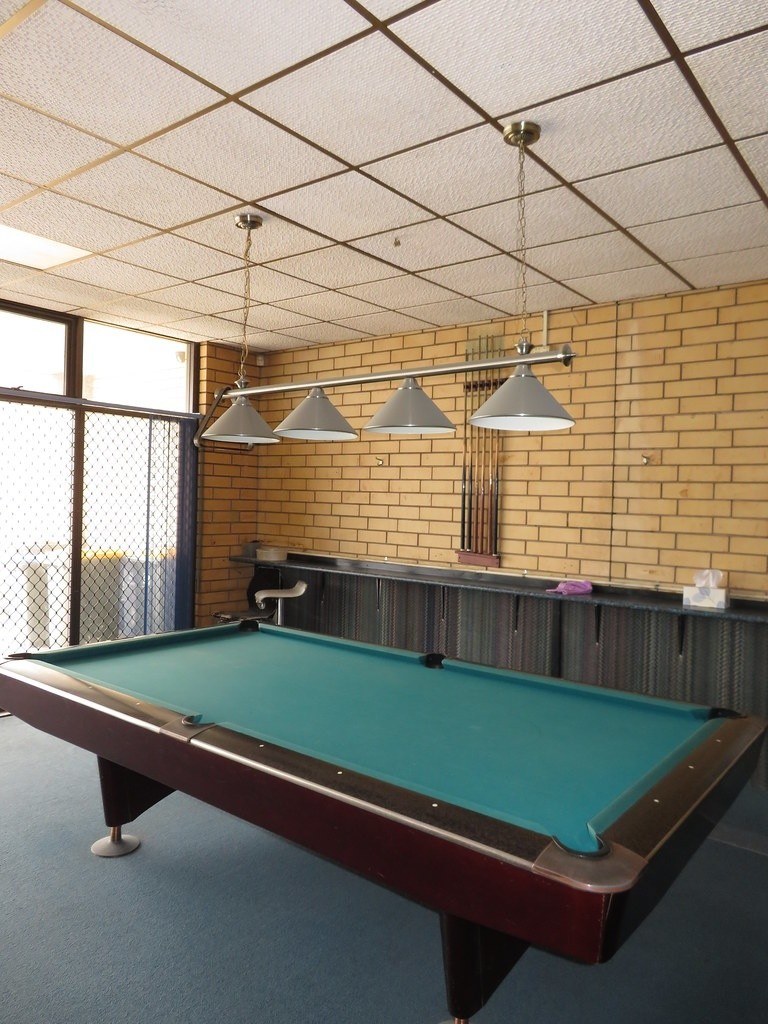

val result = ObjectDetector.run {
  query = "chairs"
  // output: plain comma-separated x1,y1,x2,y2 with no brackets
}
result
212,569,279,626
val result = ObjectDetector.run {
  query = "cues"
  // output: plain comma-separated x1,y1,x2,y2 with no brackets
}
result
461,333,504,557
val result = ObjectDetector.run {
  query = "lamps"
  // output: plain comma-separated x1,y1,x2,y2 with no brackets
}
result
200,228,281,445
468,143,577,433
272,388,359,441
362,377,457,434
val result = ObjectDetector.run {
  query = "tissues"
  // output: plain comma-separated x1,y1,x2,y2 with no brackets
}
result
683,569,731,608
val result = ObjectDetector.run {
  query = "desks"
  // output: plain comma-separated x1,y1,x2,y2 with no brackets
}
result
0,618,768,1024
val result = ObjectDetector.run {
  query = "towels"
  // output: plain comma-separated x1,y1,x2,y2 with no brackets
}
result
546,580,592,594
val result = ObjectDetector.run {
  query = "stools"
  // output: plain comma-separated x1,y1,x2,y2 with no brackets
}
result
254,580,308,625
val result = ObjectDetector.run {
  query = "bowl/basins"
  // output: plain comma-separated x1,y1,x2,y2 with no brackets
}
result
256,548,288,560
243,541,260,557
